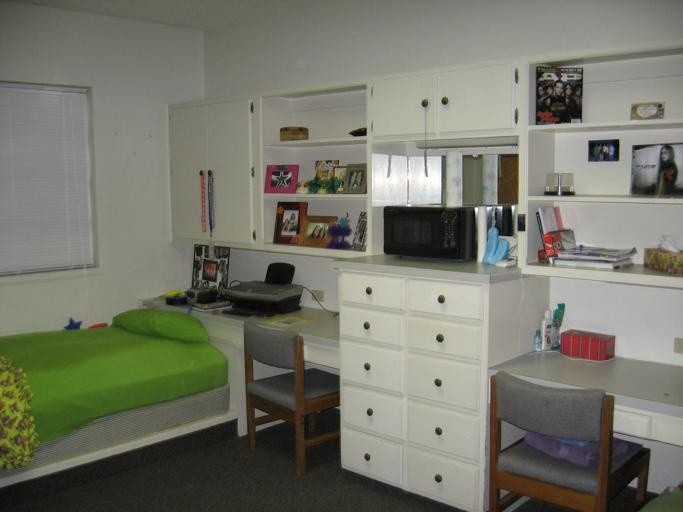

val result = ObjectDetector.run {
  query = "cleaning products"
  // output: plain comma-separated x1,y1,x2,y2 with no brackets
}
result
541,310,553,349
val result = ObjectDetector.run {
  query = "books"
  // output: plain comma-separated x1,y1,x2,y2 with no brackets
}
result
535,205,638,269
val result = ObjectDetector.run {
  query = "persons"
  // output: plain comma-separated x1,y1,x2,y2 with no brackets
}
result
348,171,358,186
285,213,298,231
352,171,363,187
282,218,290,231
651,145,678,198
536,79,582,124
306,225,321,241
595,141,611,161
315,228,325,241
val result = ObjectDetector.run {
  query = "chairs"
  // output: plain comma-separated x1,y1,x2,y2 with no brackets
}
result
489,372,650,512
242,321,339,475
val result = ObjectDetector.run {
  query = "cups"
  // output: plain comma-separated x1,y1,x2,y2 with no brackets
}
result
557,171,575,196
543,172,559,194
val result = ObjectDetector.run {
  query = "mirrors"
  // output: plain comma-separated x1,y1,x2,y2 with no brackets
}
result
406,153,519,207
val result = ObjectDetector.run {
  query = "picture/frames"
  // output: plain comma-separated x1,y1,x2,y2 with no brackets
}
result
300,215,339,247
273,201,308,247
344,164,367,194
265,164,298,193
315,159,339,181
333,166,347,192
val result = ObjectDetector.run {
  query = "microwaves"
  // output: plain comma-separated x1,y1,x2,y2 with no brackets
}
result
381,204,515,263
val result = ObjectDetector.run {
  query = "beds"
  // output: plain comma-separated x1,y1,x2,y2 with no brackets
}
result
0,325,238,487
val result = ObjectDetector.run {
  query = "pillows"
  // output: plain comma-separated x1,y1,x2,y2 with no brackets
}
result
112,308,209,344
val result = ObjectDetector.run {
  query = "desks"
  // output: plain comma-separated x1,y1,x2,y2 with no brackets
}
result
489,344,683,448
142,296,339,437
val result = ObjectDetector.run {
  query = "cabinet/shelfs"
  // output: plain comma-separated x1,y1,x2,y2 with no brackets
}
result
255,82,372,259
521,41,682,289
332,255,549,512
166,96,255,250
372,59,521,142
